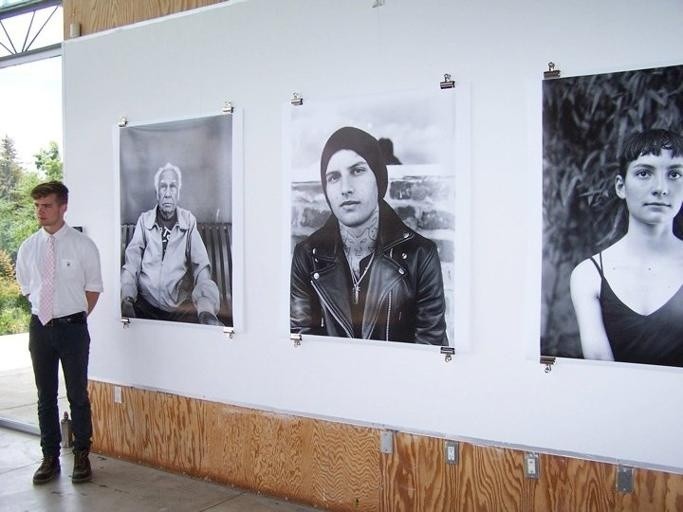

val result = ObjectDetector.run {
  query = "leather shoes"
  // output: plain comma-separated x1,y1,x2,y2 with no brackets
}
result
72,449,91,483
33,455,60,484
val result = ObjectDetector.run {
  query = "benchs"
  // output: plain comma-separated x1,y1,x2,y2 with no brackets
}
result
120,223,232,322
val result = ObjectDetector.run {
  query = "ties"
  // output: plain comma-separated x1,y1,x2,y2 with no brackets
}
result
37,237,56,326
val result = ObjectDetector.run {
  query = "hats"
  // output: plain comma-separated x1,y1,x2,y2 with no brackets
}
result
320,127,388,201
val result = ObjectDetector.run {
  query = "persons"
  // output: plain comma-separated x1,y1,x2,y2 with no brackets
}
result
123,160,227,325
288,123,448,345
12,181,102,484
568,125,681,367
